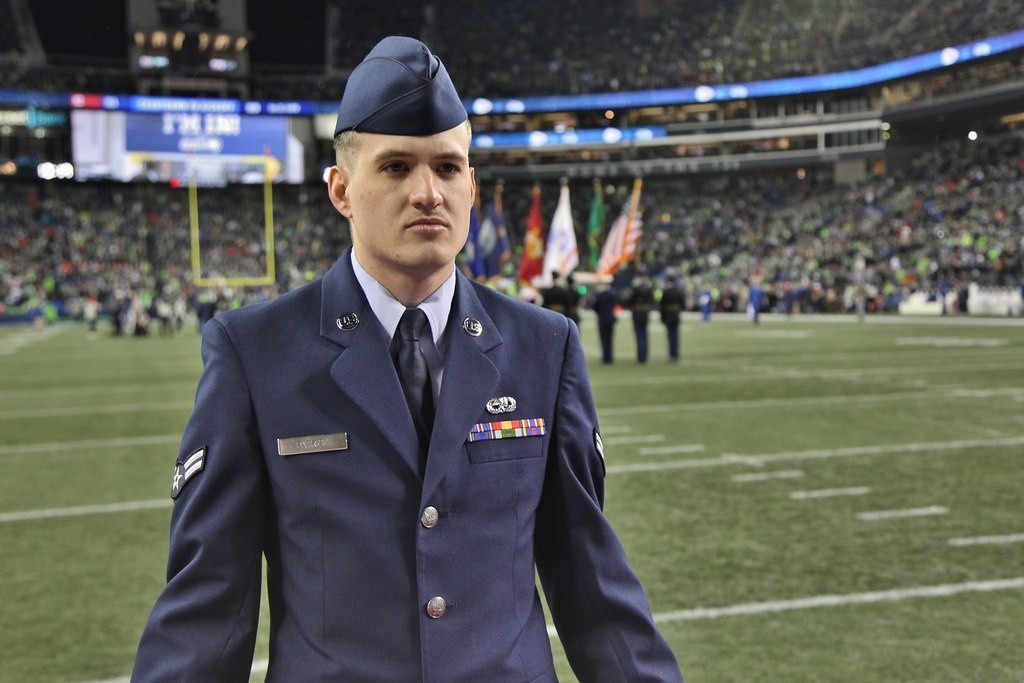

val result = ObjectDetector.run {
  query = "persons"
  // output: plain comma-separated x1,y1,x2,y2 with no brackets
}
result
536,269,580,328
1,178,350,314
646,127,1023,323
658,276,687,364
130,35,683,683
622,274,659,366
588,279,626,363
81,288,216,339
322,0,1024,115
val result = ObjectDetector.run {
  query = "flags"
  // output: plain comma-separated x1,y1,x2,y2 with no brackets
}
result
466,174,644,286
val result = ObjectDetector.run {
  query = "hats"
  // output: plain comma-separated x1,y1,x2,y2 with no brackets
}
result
334,35,468,140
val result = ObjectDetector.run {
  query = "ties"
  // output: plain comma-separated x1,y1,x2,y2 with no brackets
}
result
394,308,435,449
